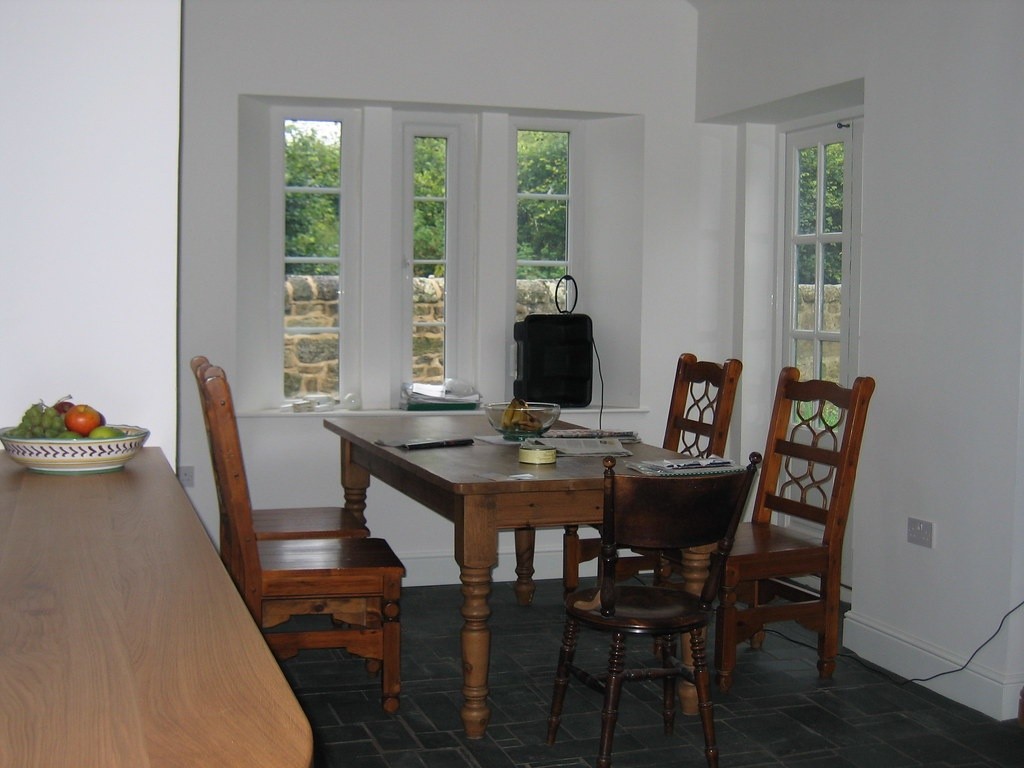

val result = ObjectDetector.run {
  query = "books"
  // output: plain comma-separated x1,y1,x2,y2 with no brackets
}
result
523,428,741,477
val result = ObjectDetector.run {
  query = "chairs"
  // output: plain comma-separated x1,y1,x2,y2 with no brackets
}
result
561,351,746,604
545,452,762,768
652,365,877,698
201,364,407,714
191,356,370,629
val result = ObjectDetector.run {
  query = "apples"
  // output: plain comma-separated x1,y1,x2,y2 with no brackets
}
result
52,401,124,439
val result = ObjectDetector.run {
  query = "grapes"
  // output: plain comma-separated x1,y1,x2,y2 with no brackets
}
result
5,399,65,439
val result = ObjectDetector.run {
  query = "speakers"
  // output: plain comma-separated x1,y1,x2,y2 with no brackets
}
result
514,314,593,408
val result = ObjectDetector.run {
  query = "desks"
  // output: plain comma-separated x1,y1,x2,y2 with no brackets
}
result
322,407,748,738
1,441,318,768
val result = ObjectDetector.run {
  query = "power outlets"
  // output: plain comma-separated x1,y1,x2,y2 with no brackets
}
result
907,517,933,550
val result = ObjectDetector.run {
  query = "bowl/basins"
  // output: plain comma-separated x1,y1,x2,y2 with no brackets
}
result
483,402,561,441
0,424,150,475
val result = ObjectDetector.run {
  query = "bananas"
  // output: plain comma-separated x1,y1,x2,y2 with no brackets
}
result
500,398,543,431
